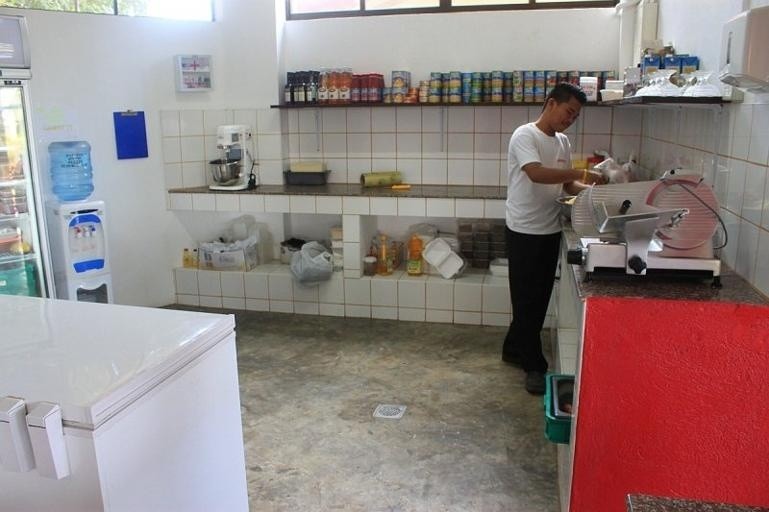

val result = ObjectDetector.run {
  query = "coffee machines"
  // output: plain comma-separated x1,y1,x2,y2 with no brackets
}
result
207,122,260,190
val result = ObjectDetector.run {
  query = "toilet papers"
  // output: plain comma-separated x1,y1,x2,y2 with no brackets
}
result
359,171,402,188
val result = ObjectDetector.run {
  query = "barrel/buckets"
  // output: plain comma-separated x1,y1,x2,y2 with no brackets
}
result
48,142,96,201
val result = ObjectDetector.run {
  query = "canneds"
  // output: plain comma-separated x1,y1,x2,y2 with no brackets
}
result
363,257,376,276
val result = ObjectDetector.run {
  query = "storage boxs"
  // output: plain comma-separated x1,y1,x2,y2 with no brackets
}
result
543,374,574,444
197,248,258,272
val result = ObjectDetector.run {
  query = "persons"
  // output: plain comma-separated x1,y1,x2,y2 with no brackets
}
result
500,83,608,395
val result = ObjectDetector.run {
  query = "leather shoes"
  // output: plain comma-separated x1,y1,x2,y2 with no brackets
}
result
502,352,547,393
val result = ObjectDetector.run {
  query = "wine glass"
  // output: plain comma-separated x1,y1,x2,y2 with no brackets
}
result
631,70,721,99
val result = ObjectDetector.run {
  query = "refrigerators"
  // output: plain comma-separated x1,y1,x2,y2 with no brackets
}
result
0,293,251,511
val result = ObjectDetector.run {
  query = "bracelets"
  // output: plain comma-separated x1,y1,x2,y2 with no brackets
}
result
583,167,591,183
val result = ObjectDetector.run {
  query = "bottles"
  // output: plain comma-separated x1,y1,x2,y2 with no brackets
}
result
407,233,423,276
282,68,384,106
